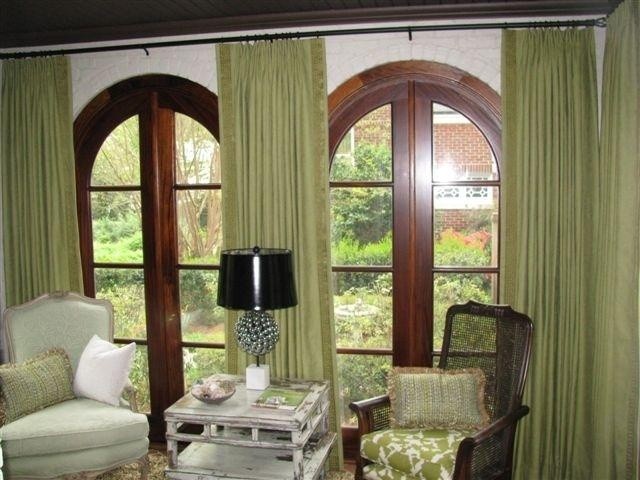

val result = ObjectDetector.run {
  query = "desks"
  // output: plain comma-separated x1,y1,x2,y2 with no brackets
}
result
162,373,338,480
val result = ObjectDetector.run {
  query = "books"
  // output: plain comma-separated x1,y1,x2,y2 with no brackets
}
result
252,379,313,412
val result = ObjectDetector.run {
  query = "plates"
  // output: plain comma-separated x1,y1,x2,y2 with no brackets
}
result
191,380,236,404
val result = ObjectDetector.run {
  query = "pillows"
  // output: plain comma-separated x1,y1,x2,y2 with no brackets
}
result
381,360,492,434
0,333,138,425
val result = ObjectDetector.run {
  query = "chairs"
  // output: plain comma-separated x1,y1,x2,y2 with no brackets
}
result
347,300,534,480
0,290,150,480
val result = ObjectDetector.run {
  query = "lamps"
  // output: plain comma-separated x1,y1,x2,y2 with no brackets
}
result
216,243,300,393
